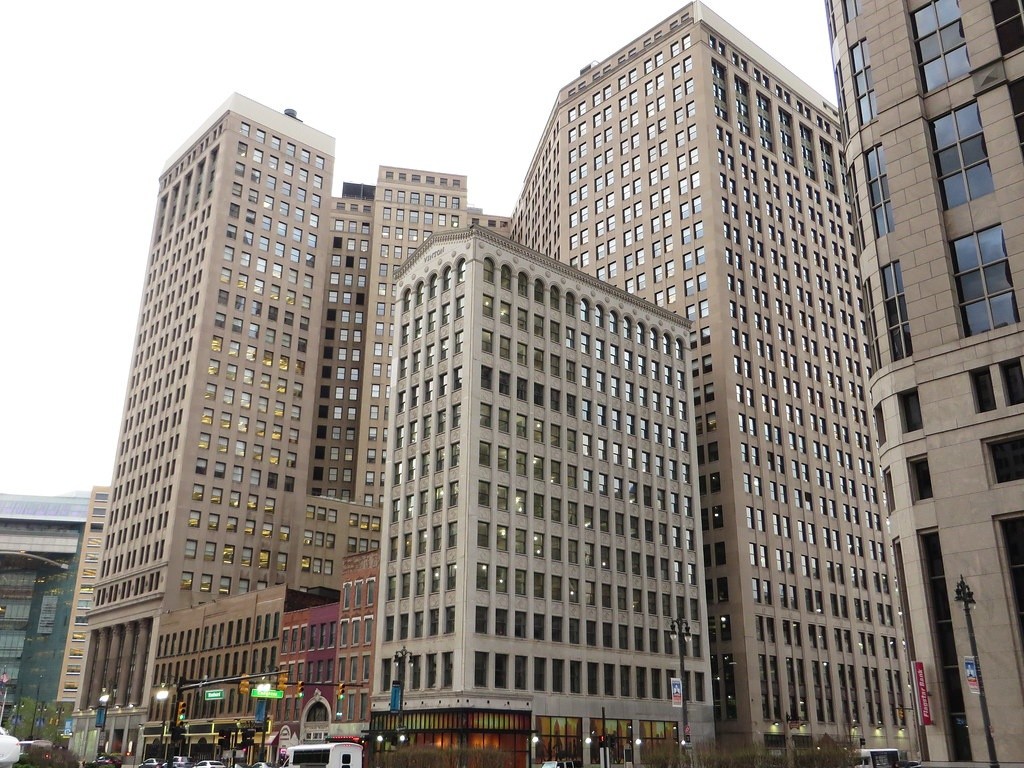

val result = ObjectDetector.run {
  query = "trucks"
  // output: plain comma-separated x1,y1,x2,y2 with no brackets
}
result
19,739,53,759
279,742,363,768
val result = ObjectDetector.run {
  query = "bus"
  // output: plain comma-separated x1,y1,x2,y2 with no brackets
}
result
848,748,901,768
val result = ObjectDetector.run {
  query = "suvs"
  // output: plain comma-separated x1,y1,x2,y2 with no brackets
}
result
162,756,194,768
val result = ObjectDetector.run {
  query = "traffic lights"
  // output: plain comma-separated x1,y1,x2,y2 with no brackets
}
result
599,735,606,747
898,708,904,720
297,681,304,697
859,737,865,745
176,701,186,726
277,669,288,691
239,674,250,694
338,683,345,700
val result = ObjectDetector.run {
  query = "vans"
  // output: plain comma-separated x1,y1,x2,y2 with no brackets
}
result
540,761,575,768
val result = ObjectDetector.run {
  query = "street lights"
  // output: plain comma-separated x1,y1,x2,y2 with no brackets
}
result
56,704,64,733
37,701,47,736
393,645,414,729
955,573,1001,768
669,613,693,753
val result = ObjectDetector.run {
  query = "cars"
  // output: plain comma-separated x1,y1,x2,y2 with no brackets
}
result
191,760,227,768
138,757,168,768
91,755,122,768
247,761,280,768
